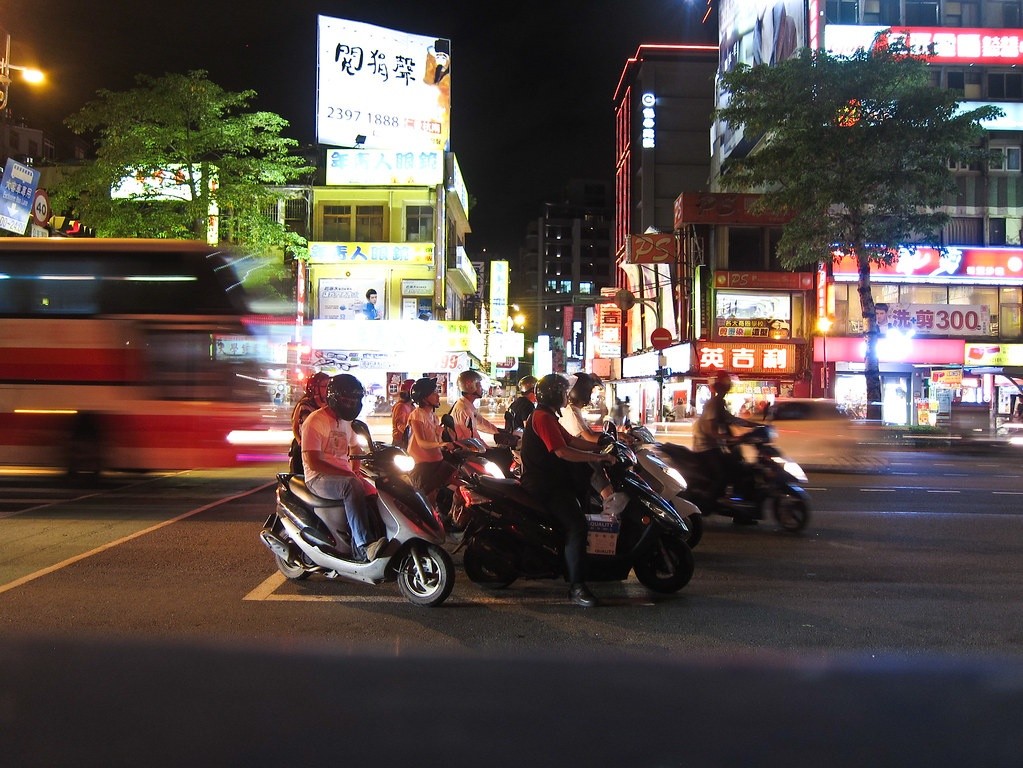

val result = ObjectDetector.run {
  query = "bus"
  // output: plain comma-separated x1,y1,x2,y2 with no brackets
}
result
0,237,275,474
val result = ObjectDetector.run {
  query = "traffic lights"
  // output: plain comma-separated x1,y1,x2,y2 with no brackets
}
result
656,370,663,378
48,215,95,238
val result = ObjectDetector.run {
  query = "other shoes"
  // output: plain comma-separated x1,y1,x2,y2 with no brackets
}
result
437,509,454,525
731,517,758,525
365,536,388,561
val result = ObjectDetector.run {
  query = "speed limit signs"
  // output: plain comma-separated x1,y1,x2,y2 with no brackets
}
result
32,189,50,227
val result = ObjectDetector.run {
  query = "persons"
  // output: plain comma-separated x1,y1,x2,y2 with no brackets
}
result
520,374,619,607
366,289,383,320
666,398,697,423
692,371,774,508
1013,395,1023,424
301,374,388,561
291,372,331,475
273,393,282,406
390,371,644,522
874,303,895,334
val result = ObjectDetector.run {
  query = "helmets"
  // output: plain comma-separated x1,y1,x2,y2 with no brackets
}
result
708,369,732,393
410,377,438,404
567,372,594,409
534,373,569,409
401,379,415,400
325,374,364,422
518,375,538,394
456,370,482,394
305,372,328,398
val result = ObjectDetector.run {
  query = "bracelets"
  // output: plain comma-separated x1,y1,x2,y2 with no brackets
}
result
438,442,443,447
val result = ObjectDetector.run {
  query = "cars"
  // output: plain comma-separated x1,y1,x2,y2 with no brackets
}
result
287,385,306,402
480,399,498,413
582,409,602,425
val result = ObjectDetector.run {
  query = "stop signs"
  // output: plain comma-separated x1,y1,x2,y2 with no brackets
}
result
651,328,672,350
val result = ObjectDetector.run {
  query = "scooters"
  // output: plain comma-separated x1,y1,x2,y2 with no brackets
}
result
620,415,703,548
650,401,811,533
287,408,525,532
454,420,694,593
259,418,456,609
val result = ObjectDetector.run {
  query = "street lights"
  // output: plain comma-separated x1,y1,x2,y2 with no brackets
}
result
816,317,830,398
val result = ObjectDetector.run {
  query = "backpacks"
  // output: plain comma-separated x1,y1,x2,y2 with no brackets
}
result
350,493,387,561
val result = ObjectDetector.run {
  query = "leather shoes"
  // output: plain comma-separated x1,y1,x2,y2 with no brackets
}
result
567,586,598,607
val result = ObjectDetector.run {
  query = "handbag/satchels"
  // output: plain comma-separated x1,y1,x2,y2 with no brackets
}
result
441,399,471,443
